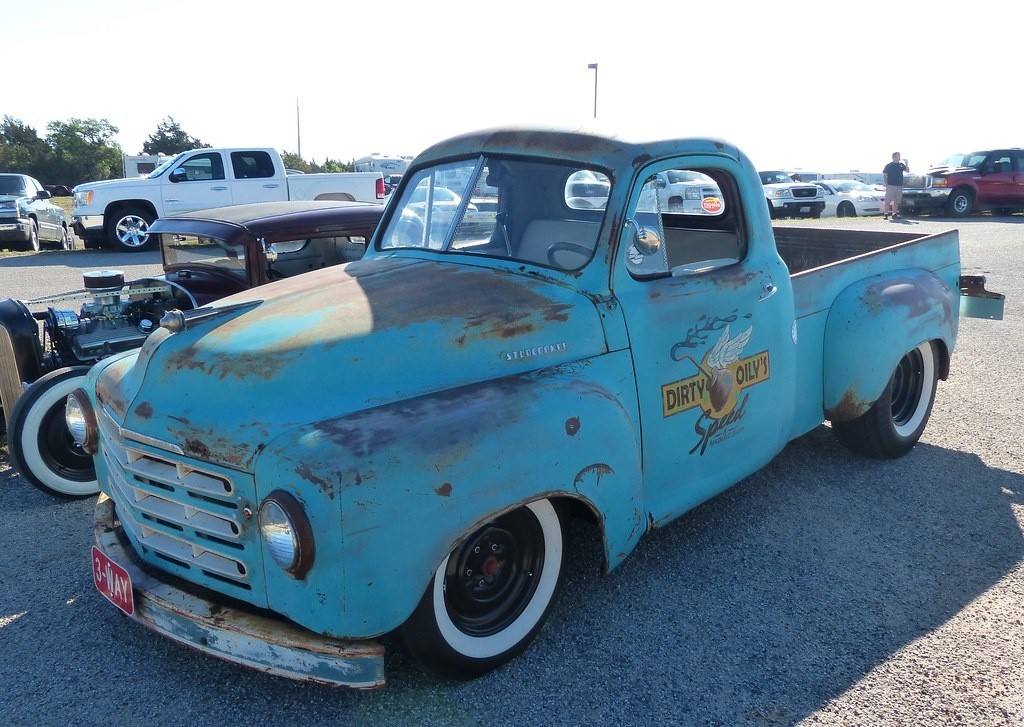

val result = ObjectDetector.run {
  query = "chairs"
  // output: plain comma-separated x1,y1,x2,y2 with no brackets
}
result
996,162,1010,172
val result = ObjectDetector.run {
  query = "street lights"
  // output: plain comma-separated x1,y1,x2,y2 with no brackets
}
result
586,61,598,120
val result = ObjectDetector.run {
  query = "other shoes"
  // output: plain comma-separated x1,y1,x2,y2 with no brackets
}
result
892,213,897,219
883,214,888,219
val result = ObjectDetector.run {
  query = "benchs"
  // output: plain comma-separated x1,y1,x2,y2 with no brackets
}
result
516,218,737,275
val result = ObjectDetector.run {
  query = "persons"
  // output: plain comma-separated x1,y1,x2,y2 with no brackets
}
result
882,152,910,220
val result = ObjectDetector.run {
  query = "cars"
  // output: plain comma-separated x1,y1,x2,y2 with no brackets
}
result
0,172,71,253
43,185,74,196
809,180,894,219
0,200,424,502
69,233,75,249
565,170,610,211
384,167,497,243
636,169,725,216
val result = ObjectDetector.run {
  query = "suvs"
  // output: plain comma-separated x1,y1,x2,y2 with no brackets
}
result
758,171,826,220
896,147,1024,217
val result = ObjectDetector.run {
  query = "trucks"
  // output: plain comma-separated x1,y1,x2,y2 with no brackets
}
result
121,150,212,181
352,153,406,179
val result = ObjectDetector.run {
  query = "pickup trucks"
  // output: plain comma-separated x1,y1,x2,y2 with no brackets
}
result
63,126,1005,693
67,148,386,252
